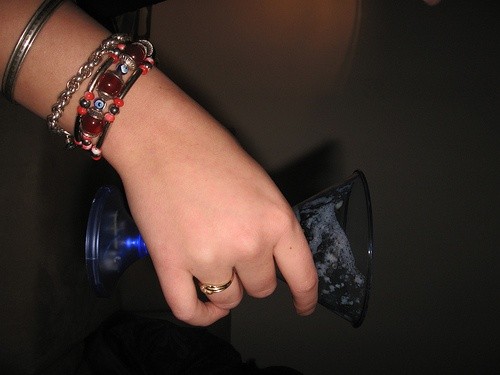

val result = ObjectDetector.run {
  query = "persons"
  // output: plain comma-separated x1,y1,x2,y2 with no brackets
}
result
0,0,320,328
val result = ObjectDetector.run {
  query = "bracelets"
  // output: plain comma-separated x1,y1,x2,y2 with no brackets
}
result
46,31,156,162
1,0,63,107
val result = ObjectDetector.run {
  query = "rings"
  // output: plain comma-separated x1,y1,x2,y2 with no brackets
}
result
198,270,237,296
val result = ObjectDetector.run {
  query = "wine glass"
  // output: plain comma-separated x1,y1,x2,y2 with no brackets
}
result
82,169,375,329
